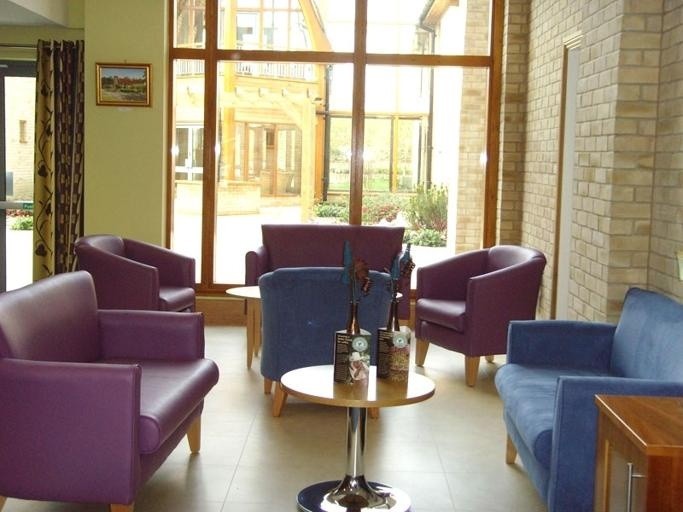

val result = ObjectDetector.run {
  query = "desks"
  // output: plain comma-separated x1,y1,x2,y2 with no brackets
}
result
280,363,436,512
226,286,263,370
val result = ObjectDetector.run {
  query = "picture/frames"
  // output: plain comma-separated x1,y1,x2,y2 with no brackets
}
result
95,62,152,109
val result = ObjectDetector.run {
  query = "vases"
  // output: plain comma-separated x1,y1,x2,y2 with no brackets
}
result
387,300,400,332
347,304,361,335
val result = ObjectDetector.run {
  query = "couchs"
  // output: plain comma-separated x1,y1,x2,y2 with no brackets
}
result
415,246,546,386
494,288,683,511
74,234,197,312
0,269,220,511
245,223,405,283
258,266,398,418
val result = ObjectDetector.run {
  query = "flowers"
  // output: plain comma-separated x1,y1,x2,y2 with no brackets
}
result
343,241,375,304
385,243,415,298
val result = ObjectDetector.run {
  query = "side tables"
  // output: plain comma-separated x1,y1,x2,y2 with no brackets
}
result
593,393,683,512
